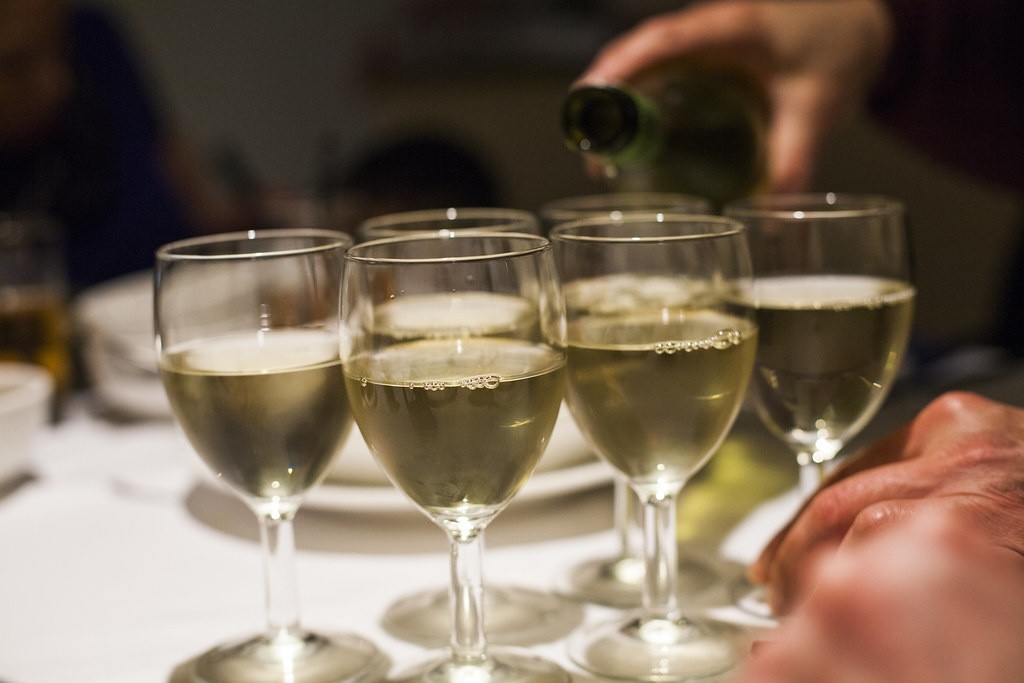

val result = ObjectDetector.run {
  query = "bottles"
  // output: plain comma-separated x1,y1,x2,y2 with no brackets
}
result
558,77,768,200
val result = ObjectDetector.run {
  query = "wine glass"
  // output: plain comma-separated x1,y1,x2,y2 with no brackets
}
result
153,226,377,683
544,195,717,604
339,232,571,683
553,214,751,683
724,193,914,620
362,211,551,631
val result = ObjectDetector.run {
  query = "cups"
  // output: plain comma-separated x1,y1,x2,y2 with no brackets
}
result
0,365,54,482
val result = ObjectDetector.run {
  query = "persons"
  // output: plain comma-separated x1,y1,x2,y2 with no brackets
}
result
739,518,1023,683
573,0,1024,618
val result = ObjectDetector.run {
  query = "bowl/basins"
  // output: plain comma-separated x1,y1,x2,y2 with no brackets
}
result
73,273,255,416
